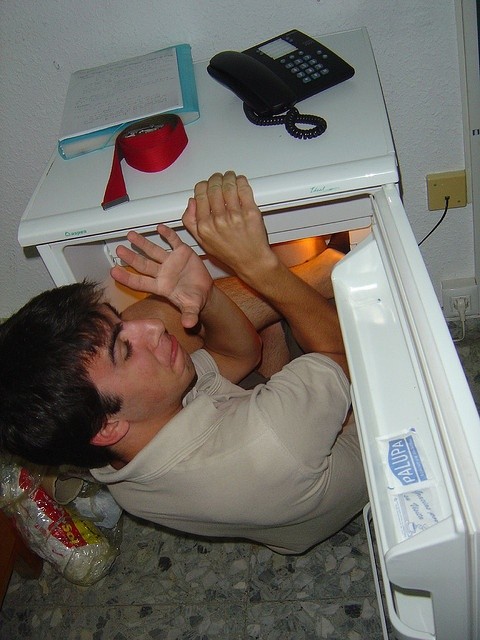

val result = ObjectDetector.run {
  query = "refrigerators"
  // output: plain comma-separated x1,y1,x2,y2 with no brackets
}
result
17,23,480,640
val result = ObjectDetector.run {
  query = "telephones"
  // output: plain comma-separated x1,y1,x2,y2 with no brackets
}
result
207,30,356,140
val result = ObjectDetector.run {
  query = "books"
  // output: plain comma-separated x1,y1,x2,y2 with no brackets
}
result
57,43,200,161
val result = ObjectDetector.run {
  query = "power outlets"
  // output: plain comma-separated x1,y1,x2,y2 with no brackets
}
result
442,277,480,319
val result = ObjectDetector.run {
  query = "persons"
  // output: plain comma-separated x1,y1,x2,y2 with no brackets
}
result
0,171,370,556
